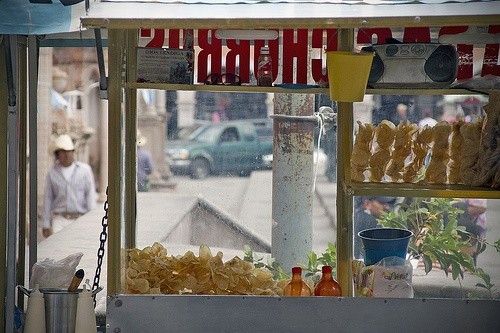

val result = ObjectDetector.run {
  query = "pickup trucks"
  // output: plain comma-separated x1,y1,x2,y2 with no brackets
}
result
163,117,273,180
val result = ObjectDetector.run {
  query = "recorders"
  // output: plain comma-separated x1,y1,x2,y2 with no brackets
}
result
361,43,459,88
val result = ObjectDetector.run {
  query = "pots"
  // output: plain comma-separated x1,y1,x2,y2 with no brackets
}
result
18,284,104,333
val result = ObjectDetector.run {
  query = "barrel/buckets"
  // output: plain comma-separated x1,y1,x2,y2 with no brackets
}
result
357,227,413,266
322,27,376,102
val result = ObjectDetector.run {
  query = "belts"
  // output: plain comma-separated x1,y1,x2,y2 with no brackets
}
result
53,211,84,220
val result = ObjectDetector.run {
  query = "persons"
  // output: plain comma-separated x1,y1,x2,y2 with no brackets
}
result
42,134,97,236
353,103,488,272
136,136,152,192
211,110,221,121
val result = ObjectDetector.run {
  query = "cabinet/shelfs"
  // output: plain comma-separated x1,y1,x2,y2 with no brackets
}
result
350,83,500,199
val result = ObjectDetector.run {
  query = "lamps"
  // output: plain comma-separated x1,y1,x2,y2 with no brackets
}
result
214,28,279,41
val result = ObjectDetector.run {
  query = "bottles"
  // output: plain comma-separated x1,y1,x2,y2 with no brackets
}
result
257,47,272,87
313,266,342,296
283,267,312,297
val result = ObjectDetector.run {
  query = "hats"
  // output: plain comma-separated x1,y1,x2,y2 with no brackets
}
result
397,103,407,110
361,195,397,205
48,134,80,157
